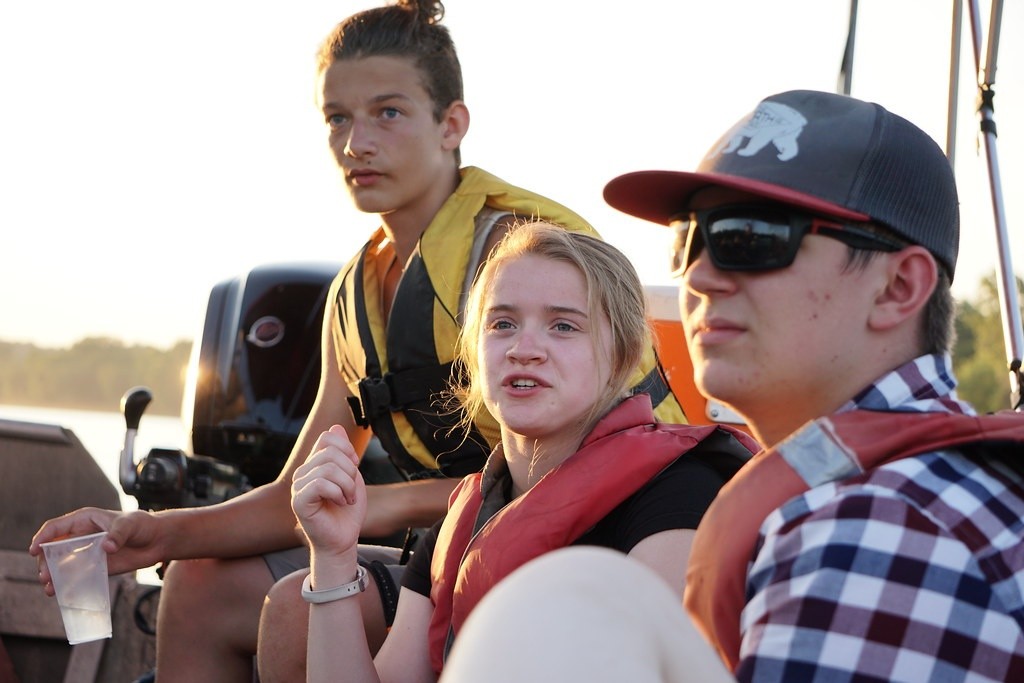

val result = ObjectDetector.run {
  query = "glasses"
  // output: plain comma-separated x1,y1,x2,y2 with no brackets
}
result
668,198,904,279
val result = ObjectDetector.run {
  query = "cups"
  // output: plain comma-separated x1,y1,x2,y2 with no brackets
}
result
38,532,113,646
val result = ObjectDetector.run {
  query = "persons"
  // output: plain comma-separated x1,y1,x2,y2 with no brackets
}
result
290,222,763,683
30,0,690,683
602,89,1024,683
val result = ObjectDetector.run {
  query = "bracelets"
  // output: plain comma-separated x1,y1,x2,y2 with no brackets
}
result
301,564,370,604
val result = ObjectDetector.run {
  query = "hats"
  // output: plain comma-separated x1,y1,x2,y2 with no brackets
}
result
603,90,960,287
